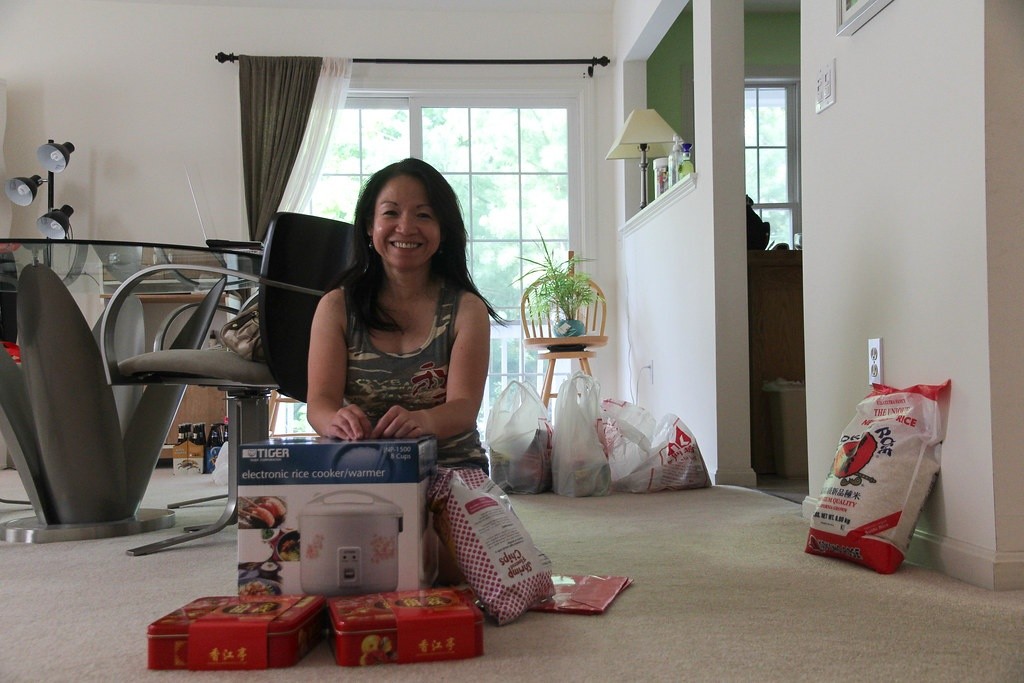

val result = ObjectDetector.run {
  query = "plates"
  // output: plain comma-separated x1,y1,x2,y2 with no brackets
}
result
238,578,288,596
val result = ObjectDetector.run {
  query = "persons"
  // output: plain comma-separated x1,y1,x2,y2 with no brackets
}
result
307,158,509,587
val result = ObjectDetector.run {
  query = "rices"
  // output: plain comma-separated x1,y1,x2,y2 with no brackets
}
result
261,561,277,570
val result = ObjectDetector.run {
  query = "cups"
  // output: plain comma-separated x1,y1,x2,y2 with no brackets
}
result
794,233,802,250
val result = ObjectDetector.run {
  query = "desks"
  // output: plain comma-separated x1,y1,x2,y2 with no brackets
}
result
0,238,262,548
516,274,607,420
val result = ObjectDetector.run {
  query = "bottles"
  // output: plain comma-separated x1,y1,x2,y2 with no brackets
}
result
177,422,228,448
653,156,669,199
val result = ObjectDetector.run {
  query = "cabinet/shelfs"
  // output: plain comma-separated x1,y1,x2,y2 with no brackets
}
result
746,252,816,474
104,281,247,462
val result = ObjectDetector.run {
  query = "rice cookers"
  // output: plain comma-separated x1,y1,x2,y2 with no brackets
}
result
296,489,404,594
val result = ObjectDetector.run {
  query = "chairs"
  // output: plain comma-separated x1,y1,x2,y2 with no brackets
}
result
101,211,357,557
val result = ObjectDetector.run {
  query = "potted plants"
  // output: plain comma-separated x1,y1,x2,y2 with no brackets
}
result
508,222,609,339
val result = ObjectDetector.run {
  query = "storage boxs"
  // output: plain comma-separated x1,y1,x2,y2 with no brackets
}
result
144,595,327,670
330,587,486,664
236,437,444,596
167,441,220,479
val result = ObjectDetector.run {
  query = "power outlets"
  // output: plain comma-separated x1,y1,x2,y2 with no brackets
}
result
867,339,885,387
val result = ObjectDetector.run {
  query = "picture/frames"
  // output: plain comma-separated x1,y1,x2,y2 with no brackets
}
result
836,0,891,37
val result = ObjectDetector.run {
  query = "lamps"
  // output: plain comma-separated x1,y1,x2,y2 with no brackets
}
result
604,108,685,211
4,140,75,240
